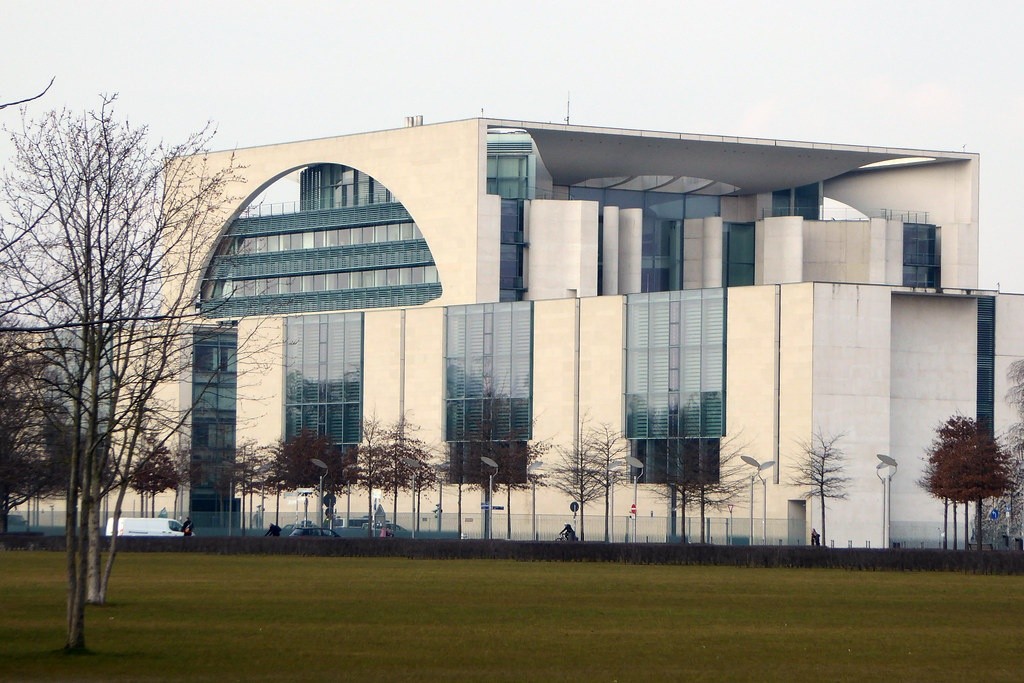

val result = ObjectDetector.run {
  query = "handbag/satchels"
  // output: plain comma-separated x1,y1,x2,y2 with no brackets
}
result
185,522,192,532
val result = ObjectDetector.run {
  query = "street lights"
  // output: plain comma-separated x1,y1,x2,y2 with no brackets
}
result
607,461,623,543
528,462,543,540
751,460,775,545
877,454,898,548
741,456,766,544
402,458,421,538
876,458,895,548
312,458,328,528
625,456,644,543
481,457,498,540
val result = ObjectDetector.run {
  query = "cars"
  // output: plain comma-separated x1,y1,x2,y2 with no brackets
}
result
362,523,407,531
289,528,340,537
334,518,368,528
7,514,30,533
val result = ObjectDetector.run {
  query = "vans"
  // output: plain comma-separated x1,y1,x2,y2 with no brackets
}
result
106,517,196,536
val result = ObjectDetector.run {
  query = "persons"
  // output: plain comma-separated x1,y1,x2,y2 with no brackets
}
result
811,528,818,544
559,523,574,540
181,517,194,536
264,522,281,537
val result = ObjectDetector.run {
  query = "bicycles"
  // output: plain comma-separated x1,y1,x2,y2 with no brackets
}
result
555,533,569,541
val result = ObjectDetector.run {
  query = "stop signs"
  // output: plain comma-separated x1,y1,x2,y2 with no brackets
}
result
632,504,636,513
